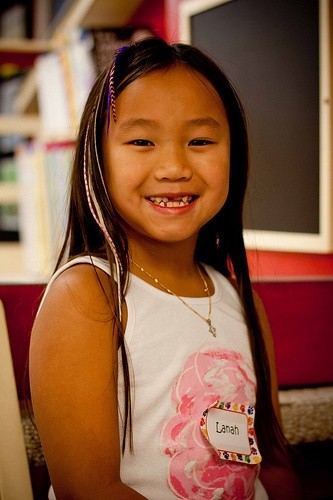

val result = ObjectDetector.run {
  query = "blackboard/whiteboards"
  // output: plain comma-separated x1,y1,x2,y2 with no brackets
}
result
178,0,331,252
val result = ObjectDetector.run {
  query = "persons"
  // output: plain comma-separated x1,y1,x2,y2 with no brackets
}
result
29,38,301,500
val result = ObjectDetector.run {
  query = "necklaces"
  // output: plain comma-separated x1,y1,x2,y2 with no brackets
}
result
126,255,218,338
